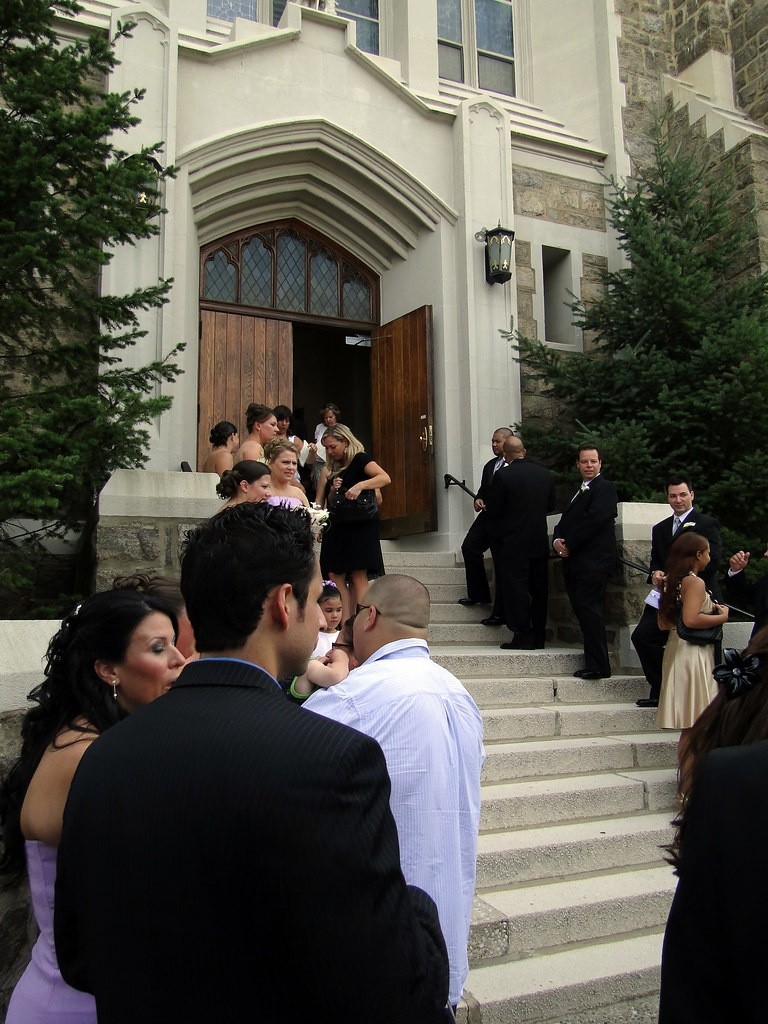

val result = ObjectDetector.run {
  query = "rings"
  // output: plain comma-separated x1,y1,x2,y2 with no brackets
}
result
559,552,562,555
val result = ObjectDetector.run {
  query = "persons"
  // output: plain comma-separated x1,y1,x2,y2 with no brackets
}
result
112,402,391,705
726,539,768,649
485,435,557,650
458,427,530,626
658,533,730,806
664,624,768,875
631,475,722,707
552,444,617,679
301,573,487,1024
55,501,456,1024
659,739,768,1024
0,590,185,1024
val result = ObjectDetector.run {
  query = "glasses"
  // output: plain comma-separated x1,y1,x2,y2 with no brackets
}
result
356,603,381,615
331,643,353,649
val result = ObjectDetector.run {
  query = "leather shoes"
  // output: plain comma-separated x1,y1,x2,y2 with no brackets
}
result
535,637,545,649
500,631,536,649
480,613,506,625
636,698,659,706
581,671,610,678
458,593,492,605
573,669,585,676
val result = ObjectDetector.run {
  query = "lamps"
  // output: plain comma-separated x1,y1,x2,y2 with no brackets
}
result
475,218,516,287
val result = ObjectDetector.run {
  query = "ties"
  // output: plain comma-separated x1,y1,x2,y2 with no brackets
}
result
673,518,679,534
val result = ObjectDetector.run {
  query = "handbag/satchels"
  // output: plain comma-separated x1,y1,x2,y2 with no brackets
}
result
327,469,377,521
675,574,722,643
